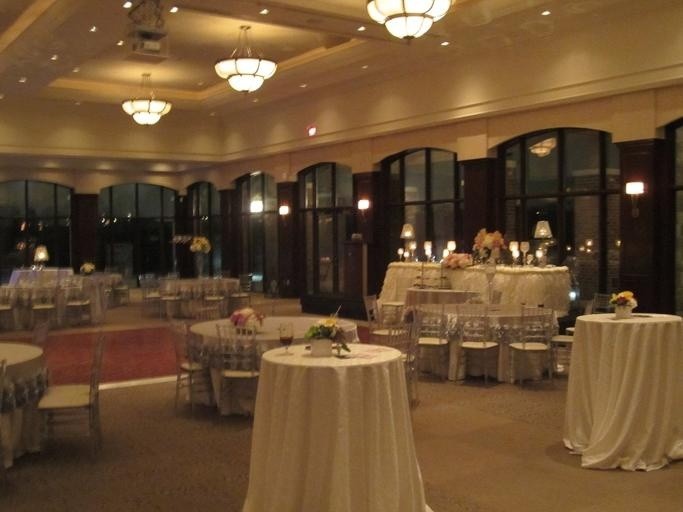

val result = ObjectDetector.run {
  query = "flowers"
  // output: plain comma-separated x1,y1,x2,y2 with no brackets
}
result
190,236,211,254
608,290,638,307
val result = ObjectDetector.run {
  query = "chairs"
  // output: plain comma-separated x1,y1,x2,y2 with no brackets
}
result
38,317,107,463
0,264,132,332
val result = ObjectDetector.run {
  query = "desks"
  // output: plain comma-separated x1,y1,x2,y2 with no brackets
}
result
243,342,434,512
562,312,683,470
0,342,46,469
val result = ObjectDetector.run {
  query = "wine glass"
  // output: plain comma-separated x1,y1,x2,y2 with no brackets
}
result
278,321,294,357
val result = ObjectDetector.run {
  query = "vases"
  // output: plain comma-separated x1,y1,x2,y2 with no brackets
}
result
197,255,209,280
614,305,632,319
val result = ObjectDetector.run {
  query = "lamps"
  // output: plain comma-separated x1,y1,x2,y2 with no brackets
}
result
529,136,558,158
395,220,553,267
121,73,173,127
626,182,644,218
366,0,452,40
34,246,49,267
214,24,279,97
